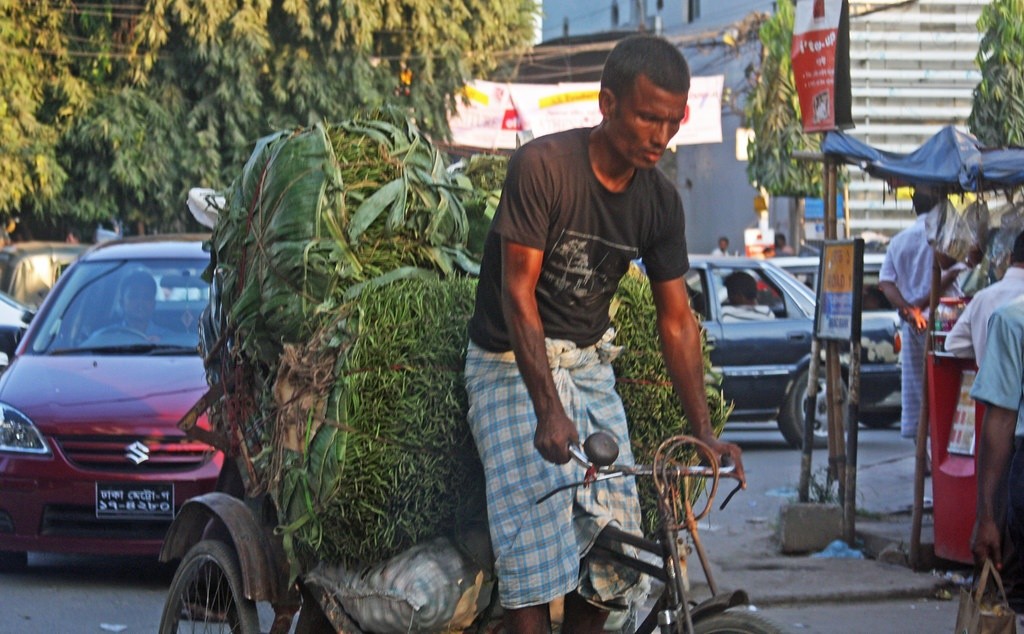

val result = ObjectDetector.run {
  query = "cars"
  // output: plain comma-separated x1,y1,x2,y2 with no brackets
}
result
0,231,235,579
632,254,903,451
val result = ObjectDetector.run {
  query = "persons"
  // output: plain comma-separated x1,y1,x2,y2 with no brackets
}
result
464,33,752,633
943,232,1024,633
749,247,777,262
775,234,793,256
720,272,777,321
75,270,197,356
710,236,735,262
878,186,967,482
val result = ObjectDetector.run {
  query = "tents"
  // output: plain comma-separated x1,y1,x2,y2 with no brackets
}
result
819,124,1024,189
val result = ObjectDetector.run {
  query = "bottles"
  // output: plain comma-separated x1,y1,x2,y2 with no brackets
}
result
935,296,973,343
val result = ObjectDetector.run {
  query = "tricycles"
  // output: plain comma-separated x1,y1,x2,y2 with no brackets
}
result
159,433,784,634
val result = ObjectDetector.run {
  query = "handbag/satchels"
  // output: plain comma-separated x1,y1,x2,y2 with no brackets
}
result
954,557,1016,633
